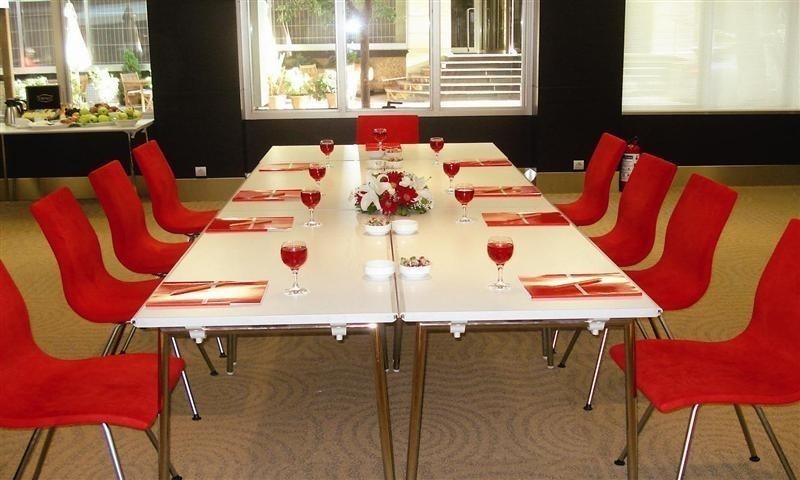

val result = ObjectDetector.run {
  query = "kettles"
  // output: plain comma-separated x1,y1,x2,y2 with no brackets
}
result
4,99,24,126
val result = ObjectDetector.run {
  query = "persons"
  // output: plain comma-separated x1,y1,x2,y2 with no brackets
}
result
24,48,40,67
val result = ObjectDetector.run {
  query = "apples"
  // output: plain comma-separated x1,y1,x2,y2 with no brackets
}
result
23,103,140,124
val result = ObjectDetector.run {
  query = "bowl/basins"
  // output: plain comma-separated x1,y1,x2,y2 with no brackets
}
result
364,260,432,281
115,120,137,127
366,160,404,169
363,220,419,236
368,150,402,157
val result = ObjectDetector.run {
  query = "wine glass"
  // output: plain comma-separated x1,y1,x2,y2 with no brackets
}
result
429,136,444,162
320,139,335,169
373,128,387,150
485,235,515,289
443,160,461,195
452,185,479,224
301,185,322,229
309,160,327,194
279,241,311,297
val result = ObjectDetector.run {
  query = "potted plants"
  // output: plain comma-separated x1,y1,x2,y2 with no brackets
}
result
264,73,337,109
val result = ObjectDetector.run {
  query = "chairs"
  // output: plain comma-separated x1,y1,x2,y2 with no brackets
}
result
605,217,799,479
130,139,222,243
551,153,679,370
120,69,153,110
1,262,190,479
355,114,420,143
28,183,220,422
86,158,228,358
552,132,628,230
556,171,741,414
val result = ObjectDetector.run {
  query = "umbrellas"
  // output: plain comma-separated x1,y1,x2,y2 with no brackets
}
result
120,5,142,54
63,0,95,106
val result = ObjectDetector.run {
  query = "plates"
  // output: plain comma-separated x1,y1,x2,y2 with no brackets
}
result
77,122,115,126
